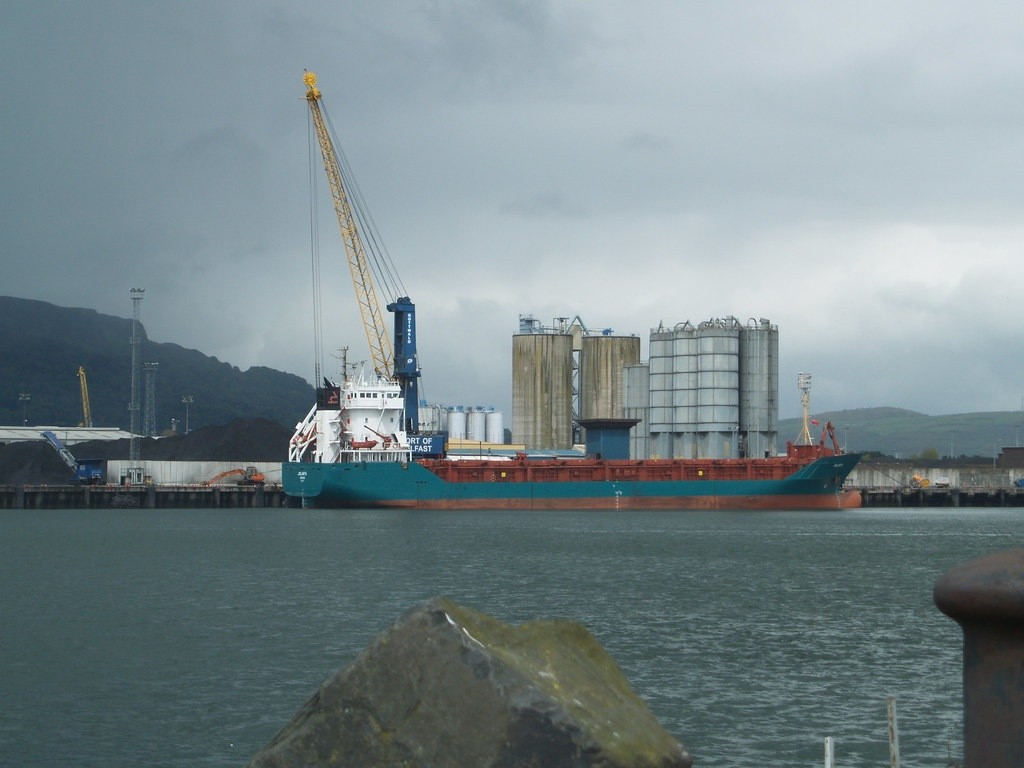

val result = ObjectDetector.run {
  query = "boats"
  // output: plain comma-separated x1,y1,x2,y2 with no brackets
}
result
279,345,867,515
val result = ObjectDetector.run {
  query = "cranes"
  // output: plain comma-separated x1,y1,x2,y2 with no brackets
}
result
298,64,400,383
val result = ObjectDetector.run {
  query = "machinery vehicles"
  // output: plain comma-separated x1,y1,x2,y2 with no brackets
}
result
199,466,266,487
40,431,103,486
